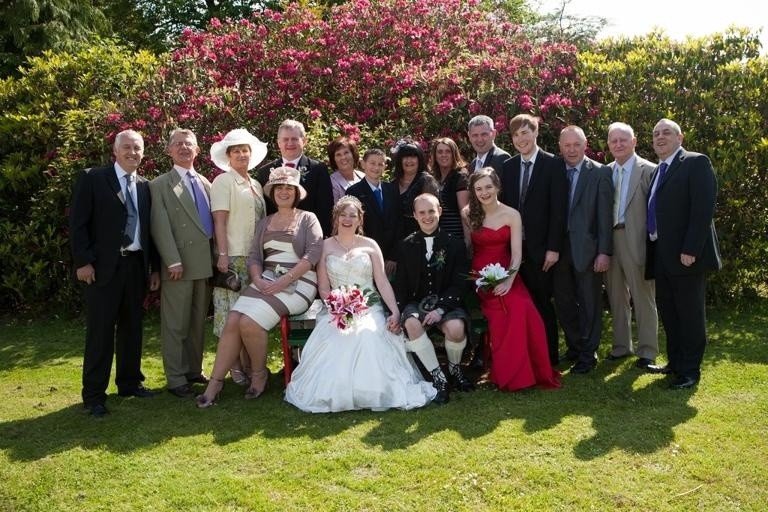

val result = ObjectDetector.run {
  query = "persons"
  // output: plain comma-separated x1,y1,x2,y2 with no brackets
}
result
258,119,334,380
283,196,436,414
461,167,561,392
329,114,568,367
150,128,214,398
195,167,324,407
72,130,160,415
210,128,268,383
399,192,474,407
560,118,721,389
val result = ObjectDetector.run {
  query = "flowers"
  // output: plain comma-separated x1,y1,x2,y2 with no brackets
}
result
459,260,523,317
325,283,381,330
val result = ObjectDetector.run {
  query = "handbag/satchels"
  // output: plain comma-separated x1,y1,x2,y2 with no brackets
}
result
206,266,242,291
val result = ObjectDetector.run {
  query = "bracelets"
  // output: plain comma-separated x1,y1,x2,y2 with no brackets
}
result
287,273,295,281
219,252,228,256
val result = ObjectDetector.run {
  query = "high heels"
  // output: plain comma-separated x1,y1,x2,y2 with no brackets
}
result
230,368,250,387
196,377,224,409
244,366,272,400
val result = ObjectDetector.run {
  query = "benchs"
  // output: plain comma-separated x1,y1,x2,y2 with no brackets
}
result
280,295,488,391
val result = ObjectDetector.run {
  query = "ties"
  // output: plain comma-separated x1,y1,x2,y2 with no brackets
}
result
612,167,624,228
477,159,483,170
121,174,139,249
646,162,669,236
285,163,295,169
520,161,533,213
375,190,384,211
186,170,214,239
567,169,576,193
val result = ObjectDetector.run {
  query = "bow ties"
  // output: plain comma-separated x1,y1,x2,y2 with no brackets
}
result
424,237,436,262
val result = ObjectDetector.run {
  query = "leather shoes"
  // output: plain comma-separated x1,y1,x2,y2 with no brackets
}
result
609,349,627,358
190,373,213,384
671,374,699,388
85,401,108,417
455,373,473,393
434,379,450,404
647,361,675,373
637,358,656,370
118,385,157,398
168,384,196,399
565,347,598,375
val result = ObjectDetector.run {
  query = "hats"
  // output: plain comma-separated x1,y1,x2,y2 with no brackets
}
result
263,166,307,202
210,128,269,173
